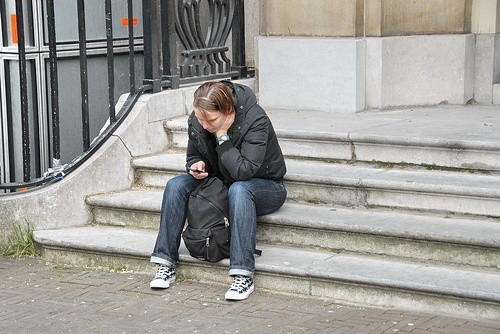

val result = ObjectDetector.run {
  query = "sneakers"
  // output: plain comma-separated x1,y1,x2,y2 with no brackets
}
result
150,264,176,288
225,275,254,300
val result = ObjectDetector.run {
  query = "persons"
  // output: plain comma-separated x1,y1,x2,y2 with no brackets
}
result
149,81,287,301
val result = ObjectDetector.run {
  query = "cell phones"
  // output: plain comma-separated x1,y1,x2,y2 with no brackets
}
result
186,168,207,174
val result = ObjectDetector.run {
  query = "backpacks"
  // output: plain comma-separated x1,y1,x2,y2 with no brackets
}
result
182,176,230,263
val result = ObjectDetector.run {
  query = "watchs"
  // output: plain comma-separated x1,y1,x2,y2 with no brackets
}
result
215,135,230,143
